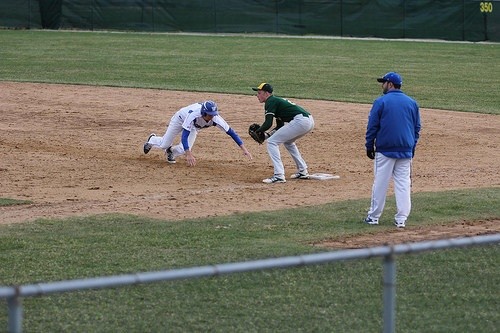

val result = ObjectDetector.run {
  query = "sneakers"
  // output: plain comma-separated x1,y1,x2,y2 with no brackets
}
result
262,175,286,183
165,146,176,164
144,133,156,154
290,169,309,179
393,220,405,228
363,215,378,225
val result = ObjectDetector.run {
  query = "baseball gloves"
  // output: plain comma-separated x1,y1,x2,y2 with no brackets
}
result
249,123,265,144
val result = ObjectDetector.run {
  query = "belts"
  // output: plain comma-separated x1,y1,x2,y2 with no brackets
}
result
303,114,308,117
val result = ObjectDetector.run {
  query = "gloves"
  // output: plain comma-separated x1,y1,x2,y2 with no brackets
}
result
367,147,375,159
412,150,414,158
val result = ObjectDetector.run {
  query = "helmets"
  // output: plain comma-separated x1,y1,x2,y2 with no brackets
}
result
201,101,218,117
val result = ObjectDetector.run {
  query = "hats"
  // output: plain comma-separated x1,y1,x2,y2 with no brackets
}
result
377,72,402,85
252,83,273,93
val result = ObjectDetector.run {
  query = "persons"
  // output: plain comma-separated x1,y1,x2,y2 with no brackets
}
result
143,101,253,166
252,83,314,182
364,72,422,227
